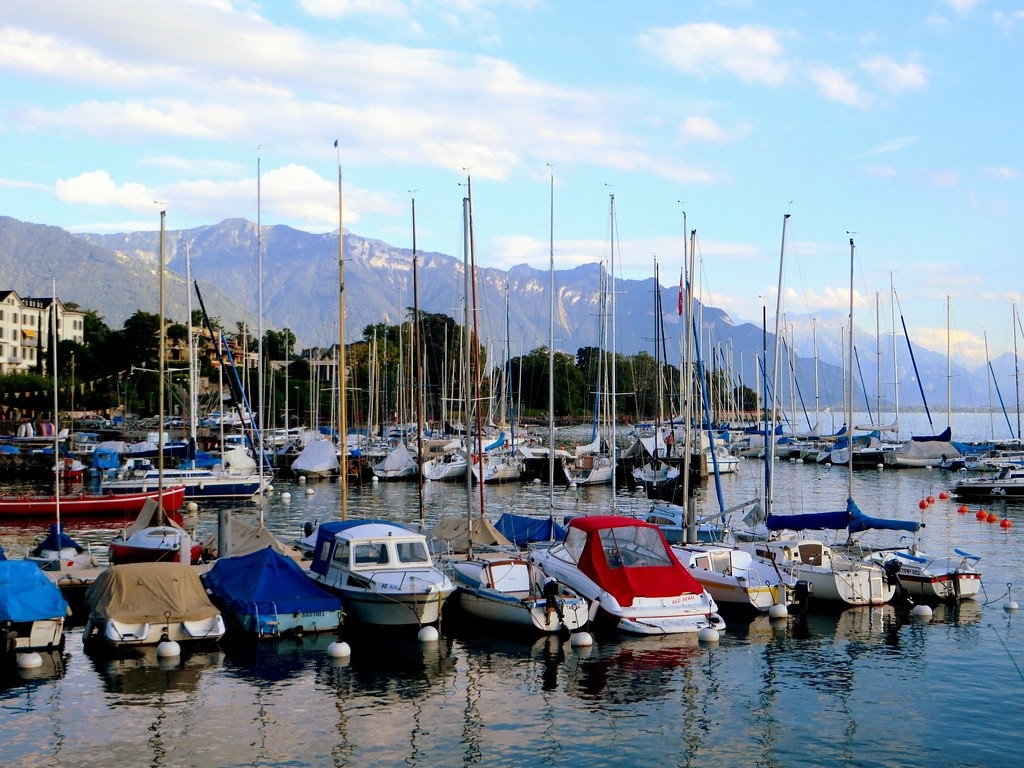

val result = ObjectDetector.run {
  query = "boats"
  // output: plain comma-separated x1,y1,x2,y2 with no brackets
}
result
0,545,75,656
199,541,346,641
527,514,727,635
81,562,226,646
299,515,458,629
736,534,905,606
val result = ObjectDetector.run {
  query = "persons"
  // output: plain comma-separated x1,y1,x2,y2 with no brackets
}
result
664,433,674,458
36,411,43,420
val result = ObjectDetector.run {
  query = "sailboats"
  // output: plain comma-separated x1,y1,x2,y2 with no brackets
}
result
426,196,590,636
664,229,816,615
24,269,96,573
105,200,204,565
831,225,985,600
0,129,1023,559
